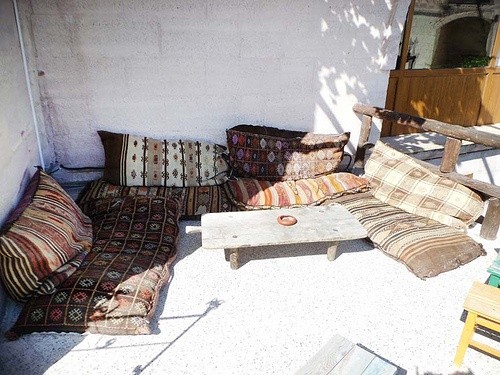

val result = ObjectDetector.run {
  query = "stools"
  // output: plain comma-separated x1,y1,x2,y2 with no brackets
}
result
455,280,500,363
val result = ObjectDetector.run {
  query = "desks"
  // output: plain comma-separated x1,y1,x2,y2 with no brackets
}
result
201,206,367,268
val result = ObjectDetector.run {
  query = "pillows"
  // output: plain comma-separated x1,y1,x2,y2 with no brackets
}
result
325,188,486,278
362,141,485,227
224,172,369,210
75,179,226,219
0,166,91,302
5,194,183,338
225,124,350,181
97,130,231,186
